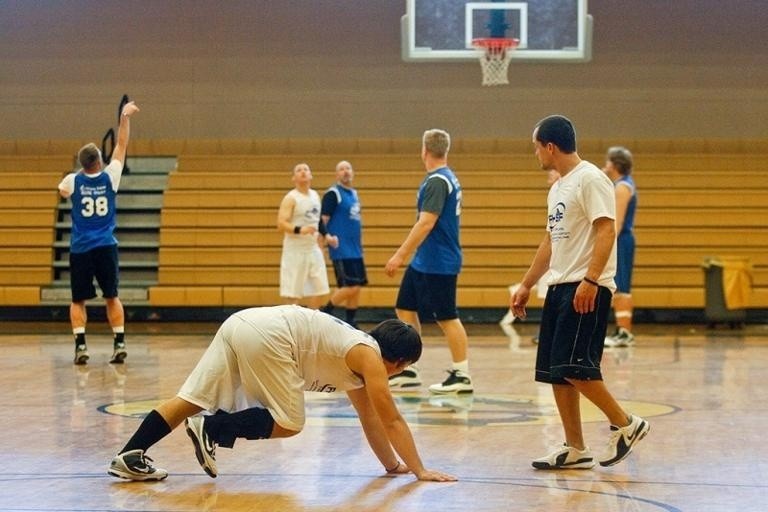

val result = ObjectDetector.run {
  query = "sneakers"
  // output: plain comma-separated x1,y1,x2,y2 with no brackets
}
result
107,449,172,484
599,330,635,348
109,347,127,367
531,447,600,470
428,370,474,397
600,414,651,467
184,414,227,479
74,346,90,366
387,369,421,390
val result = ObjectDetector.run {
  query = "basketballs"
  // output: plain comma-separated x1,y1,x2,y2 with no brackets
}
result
483,45,508,68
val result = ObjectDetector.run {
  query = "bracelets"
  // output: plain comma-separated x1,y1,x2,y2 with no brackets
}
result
385,461,401,473
120,111,130,118
584,277,598,286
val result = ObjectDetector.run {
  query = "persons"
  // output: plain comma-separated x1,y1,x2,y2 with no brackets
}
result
57,102,140,364
509,114,651,470
532,170,560,346
276,163,339,309
108,305,459,482
601,146,638,348
318,161,369,331
383,128,474,392
